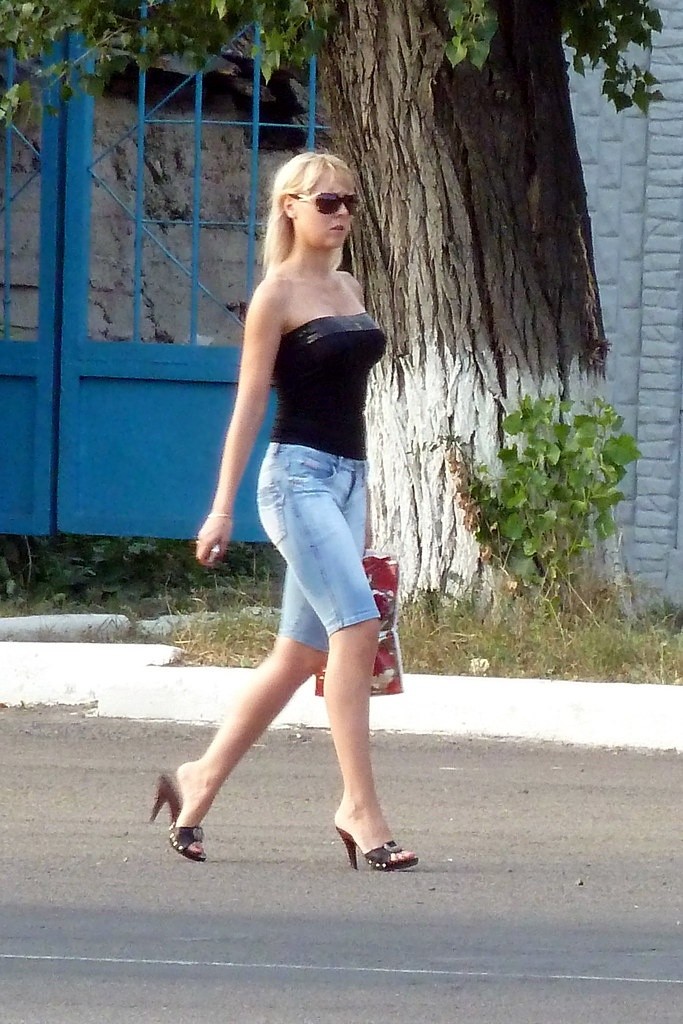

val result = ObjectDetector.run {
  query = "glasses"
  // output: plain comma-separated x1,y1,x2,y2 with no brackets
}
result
289,193,359,215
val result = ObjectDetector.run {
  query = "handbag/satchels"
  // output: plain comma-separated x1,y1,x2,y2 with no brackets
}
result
315,548,402,697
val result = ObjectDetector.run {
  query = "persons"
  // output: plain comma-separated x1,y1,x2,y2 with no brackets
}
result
149,153,419,874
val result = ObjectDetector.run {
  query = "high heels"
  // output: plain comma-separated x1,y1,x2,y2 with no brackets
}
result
336,826,419,871
149,774,207,862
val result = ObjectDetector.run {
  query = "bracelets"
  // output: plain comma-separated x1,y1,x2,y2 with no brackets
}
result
208,514,231,518
